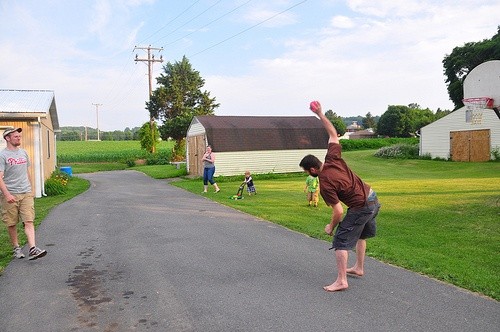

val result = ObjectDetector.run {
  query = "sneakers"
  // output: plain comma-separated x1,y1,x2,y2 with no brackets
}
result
13,247,25,259
28,247,47,260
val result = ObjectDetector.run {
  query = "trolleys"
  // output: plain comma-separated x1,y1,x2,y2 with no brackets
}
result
230,182,246,199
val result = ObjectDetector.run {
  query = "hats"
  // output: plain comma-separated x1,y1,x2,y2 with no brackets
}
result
3,127,22,139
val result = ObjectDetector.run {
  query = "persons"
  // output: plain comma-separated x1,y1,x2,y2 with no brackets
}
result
299,101,380,291
304,174,320,207
0,128,47,260
201,145,220,194
243,171,257,196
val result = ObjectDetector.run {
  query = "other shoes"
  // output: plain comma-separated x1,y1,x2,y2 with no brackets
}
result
201,191,207,194
213,189,220,193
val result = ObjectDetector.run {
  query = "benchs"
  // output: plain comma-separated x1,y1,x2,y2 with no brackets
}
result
168,162,186,169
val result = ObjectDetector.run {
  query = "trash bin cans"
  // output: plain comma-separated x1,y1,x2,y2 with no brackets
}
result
60,167,72,177
